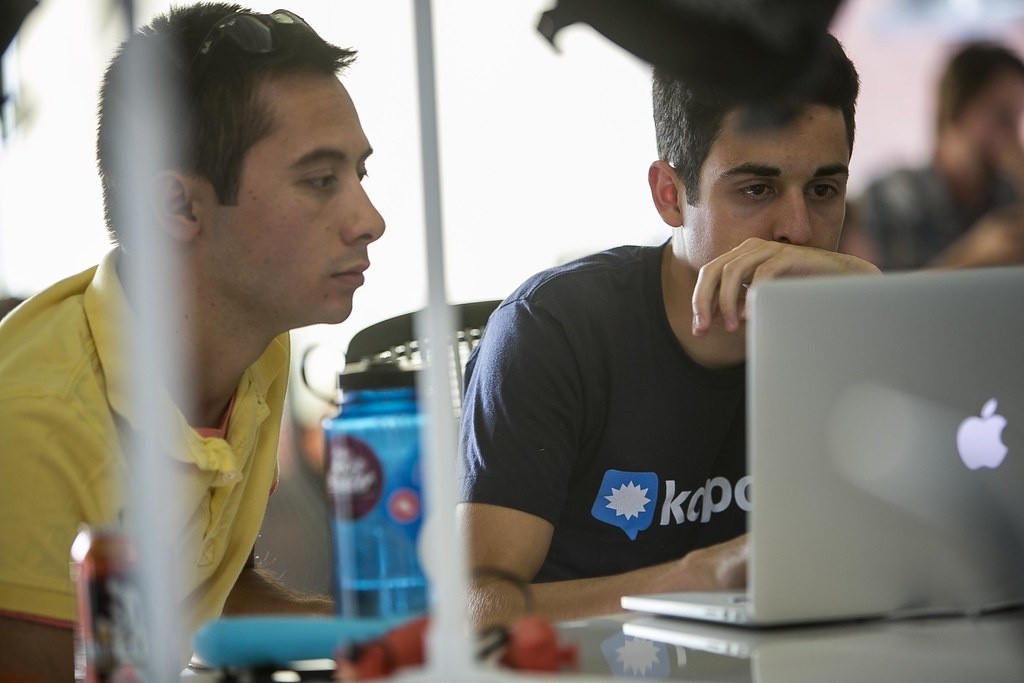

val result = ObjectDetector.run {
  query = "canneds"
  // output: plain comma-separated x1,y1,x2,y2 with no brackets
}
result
69,522,163,683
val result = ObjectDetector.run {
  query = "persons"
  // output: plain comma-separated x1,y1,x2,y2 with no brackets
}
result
439,16,881,620
0,2,386,683
842,40,1024,271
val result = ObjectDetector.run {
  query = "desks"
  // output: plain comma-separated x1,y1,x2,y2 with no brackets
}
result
552,612,1024,683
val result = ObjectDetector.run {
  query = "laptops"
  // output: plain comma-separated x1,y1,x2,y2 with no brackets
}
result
620,266,1024,625
619,616,1023,683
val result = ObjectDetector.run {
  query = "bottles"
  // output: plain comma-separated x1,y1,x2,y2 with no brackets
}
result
300,342,427,620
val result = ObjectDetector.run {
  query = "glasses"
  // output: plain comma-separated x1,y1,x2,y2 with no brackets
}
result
181,9,315,100
